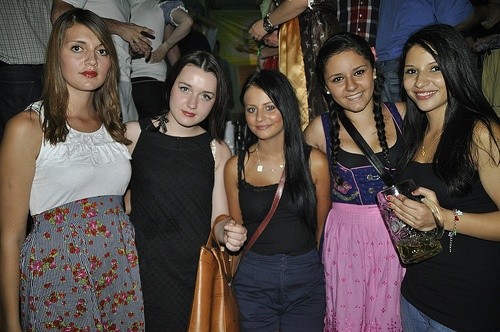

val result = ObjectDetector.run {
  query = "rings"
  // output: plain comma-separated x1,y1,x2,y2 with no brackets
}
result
241,242,243,246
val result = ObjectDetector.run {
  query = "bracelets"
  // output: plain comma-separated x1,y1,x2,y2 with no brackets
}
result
448,209,462,252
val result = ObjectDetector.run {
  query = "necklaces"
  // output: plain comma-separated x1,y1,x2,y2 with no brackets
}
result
255,144,284,173
420,128,443,156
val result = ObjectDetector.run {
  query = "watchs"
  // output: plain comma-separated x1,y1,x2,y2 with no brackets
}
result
263,13,278,34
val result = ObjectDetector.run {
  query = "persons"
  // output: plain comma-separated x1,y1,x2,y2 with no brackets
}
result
0,0,500,332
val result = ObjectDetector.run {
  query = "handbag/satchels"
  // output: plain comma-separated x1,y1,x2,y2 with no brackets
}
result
187,215,243,332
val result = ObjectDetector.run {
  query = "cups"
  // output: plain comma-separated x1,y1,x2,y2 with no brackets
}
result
377,179,445,264
